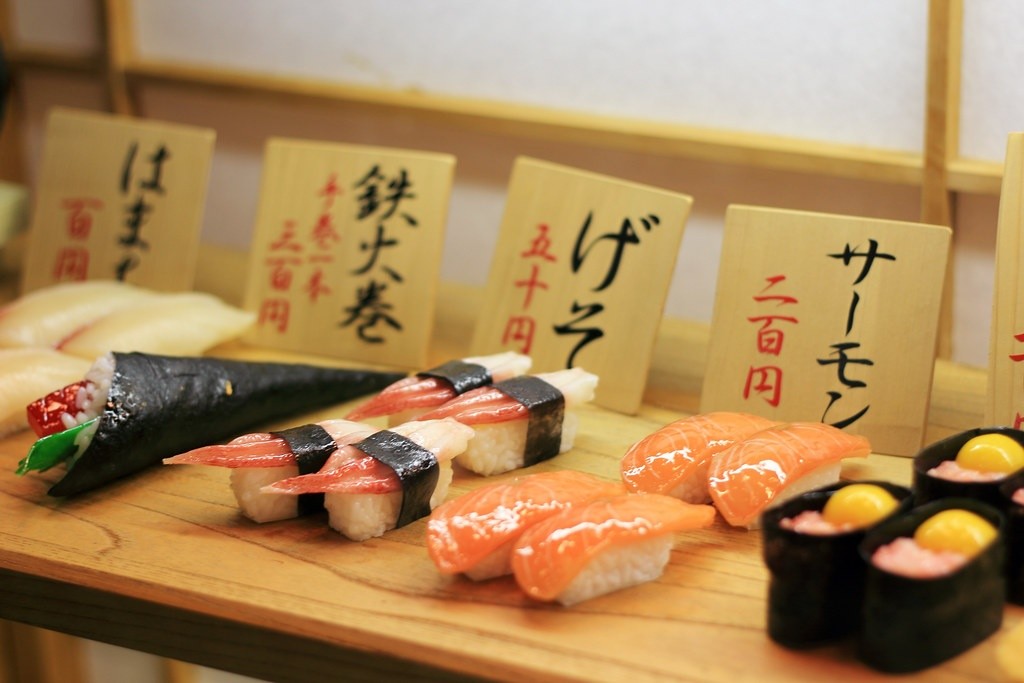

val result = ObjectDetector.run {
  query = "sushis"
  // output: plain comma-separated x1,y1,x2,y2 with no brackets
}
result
0,278,873,605
760,425,1024,675
